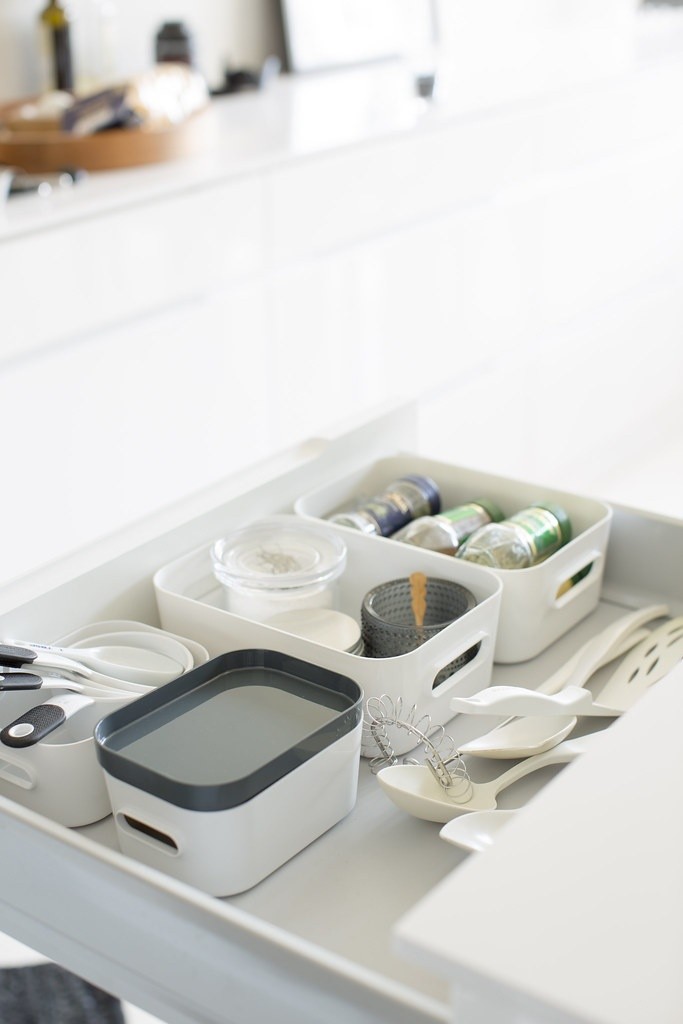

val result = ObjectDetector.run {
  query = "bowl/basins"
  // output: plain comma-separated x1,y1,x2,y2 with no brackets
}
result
256,610,368,685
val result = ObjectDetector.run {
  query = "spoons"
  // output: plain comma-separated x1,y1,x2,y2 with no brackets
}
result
0,636,183,696
440,806,518,849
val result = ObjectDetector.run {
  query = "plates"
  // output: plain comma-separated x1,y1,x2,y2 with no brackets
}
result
212,519,348,600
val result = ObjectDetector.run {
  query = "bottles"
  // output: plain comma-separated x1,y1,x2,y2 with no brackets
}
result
326,475,440,535
390,499,504,556
459,506,569,569
